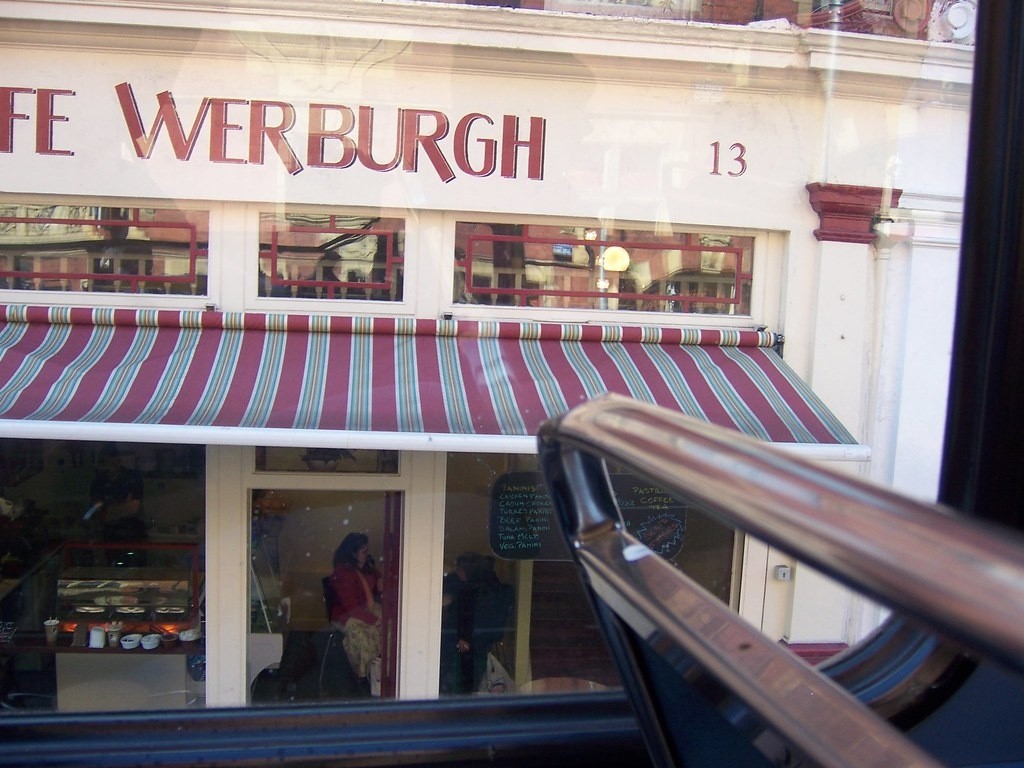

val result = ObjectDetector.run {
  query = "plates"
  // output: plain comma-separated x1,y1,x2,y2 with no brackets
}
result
156,610,184,613
76,609,105,613
116,609,144,613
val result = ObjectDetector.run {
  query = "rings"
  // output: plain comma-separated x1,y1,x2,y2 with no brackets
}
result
466,647,467,648
464,645,465,647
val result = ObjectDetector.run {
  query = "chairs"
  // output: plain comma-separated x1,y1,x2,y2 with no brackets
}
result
536,395,1023,767
469,584,512,692
317,575,345,687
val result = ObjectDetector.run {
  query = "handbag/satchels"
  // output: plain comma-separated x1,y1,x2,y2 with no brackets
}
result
479,652,515,695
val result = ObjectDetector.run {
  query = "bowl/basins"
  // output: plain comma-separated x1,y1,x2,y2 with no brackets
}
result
121,634,142,649
162,634,180,650
141,635,161,649
180,630,201,649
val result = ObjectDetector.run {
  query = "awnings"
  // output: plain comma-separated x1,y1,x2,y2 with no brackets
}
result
0,303,875,464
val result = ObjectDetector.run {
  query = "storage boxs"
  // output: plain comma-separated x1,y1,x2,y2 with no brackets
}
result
144,522,201,543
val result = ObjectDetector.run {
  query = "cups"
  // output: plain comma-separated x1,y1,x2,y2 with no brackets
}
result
88,627,105,648
44,620,60,645
108,626,122,648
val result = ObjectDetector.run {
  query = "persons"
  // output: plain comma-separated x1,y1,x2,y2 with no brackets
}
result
443,552,506,694
258,249,403,302
88,450,149,555
454,247,477,305
328,532,382,678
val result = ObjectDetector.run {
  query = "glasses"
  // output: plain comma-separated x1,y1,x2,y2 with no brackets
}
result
358,549,370,554
